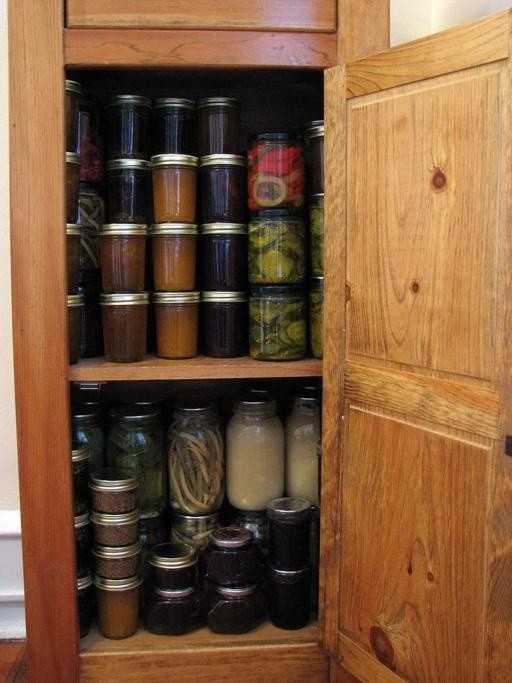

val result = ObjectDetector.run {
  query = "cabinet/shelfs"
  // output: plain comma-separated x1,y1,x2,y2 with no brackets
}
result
8,0,512,683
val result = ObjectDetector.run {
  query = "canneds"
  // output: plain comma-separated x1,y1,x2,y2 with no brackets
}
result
64,80,325,365
70,379,322,639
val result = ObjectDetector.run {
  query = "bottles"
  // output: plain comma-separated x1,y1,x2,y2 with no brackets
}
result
110,157,245,221
76,107,103,359
308,120,326,357
289,388,320,506
71,443,92,635
108,92,239,152
101,224,246,292
266,496,316,629
229,388,287,511
204,526,269,634
90,467,140,639
77,397,106,494
100,294,250,357
111,399,164,511
163,402,228,514
64,78,85,359
245,128,309,362
141,540,202,636
